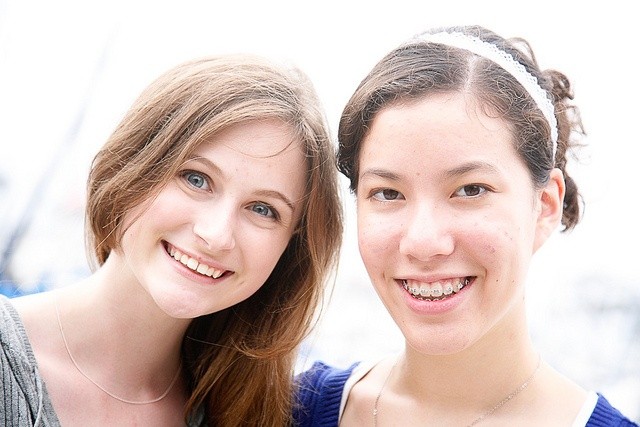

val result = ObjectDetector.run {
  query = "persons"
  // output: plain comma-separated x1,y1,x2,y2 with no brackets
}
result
283,22,640,427
0,55,344,427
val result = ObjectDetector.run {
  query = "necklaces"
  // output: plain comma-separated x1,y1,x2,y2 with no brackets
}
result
371,358,537,427
53,284,182,406
